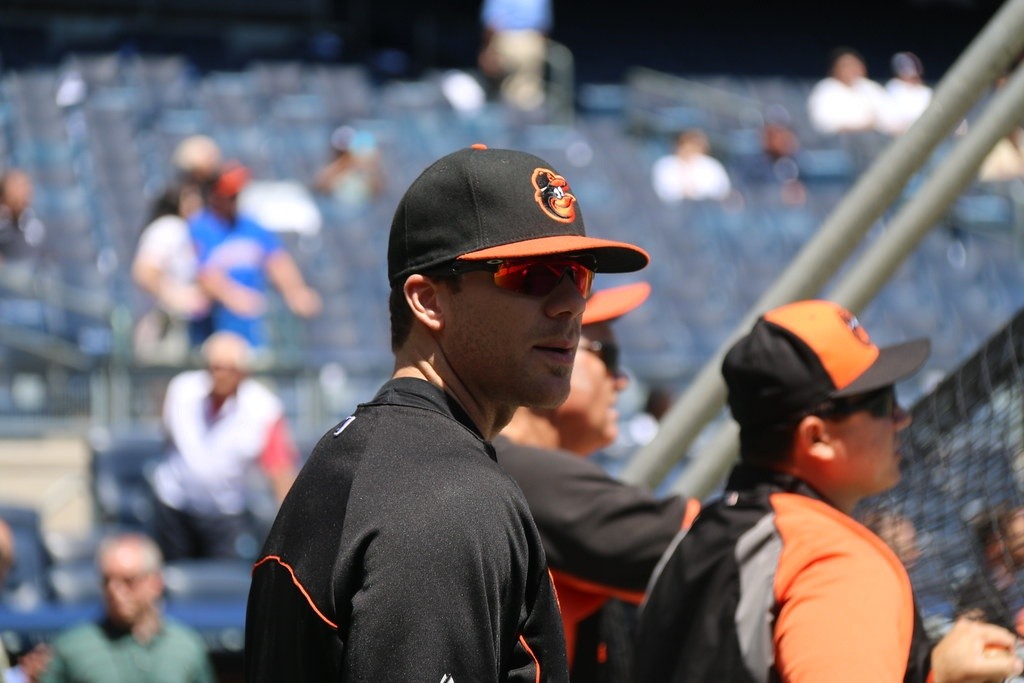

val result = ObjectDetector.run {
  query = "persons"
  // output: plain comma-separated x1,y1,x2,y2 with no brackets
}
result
954,502,1023,637
0,169,38,256
477,0,552,109
977,77,1024,182
634,297,1024,683
809,50,903,139
241,140,650,683
324,122,383,201
33,534,213,683
145,330,297,564
129,134,321,370
864,512,924,575
652,127,731,207
489,280,699,683
633,385,670,444
887,50,968,134
748,123,806,195
0,517,51,683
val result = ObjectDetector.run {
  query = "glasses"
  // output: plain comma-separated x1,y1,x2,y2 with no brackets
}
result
572,341,619,371
425,255,593,301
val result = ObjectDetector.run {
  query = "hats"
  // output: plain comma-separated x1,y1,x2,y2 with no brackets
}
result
580,280,651,326
720,302,930,424
388,144,648,284
